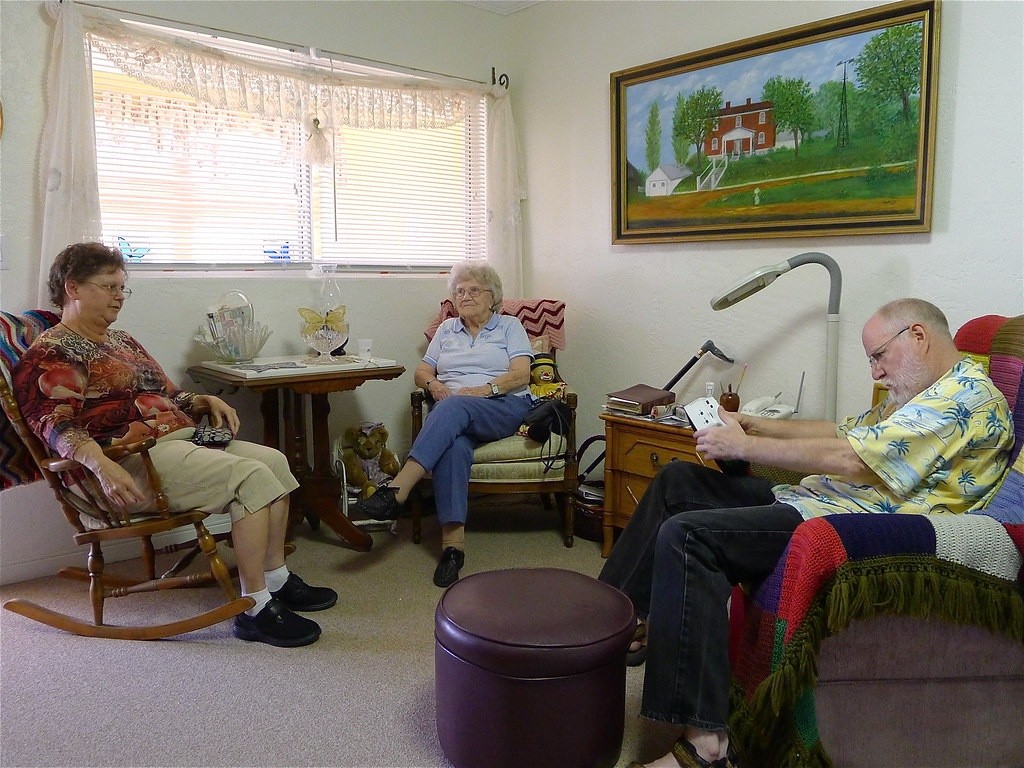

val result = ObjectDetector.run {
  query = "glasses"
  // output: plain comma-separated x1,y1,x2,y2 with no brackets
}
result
868,327,911,365
452,287,492,298
75,281,133,299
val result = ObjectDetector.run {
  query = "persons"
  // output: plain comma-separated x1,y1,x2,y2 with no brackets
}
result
12,242,338,647
597,299,1016,768
357,259,534,588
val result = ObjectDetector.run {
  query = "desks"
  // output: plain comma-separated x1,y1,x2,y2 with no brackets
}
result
186,366,407,552
599,414,720,559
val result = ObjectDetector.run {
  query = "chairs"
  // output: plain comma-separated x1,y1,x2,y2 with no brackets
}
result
0,310,296,639
411,299,577,548
732,315,1024,768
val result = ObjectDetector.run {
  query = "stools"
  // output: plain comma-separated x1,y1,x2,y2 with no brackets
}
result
436,567,635,768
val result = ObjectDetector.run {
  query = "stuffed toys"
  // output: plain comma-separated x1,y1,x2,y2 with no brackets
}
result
338,421,400,500
519,352,577,446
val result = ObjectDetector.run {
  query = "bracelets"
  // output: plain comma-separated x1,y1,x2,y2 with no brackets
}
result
424,378,436,389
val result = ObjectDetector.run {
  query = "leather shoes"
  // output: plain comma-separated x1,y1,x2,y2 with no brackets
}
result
353,486,399,522
433,546,465,587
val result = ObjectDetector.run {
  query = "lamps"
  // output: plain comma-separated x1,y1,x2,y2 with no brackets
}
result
711,252,841,419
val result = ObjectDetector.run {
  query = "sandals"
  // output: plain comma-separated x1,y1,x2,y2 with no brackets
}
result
628,616,647,667
625,736,736,768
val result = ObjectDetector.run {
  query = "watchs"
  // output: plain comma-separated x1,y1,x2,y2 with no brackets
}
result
487,381,499,394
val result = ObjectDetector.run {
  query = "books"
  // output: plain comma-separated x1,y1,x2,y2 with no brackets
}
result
683,397,752,476
601,383,675,415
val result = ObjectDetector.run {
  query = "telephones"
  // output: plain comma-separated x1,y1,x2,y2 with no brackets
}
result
740,370,806,419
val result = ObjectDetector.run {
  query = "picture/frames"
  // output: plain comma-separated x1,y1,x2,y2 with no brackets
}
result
610,1,940,244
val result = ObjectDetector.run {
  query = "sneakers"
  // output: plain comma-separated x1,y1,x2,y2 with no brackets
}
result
270,571,338,610
234,599,321,647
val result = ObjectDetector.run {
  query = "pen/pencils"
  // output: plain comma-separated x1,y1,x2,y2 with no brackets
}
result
719,382,724,393
728,384,731,392
734,363,748,393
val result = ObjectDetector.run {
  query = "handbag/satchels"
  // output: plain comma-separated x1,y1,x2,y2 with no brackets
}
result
192,424,232,447
525,399,570,434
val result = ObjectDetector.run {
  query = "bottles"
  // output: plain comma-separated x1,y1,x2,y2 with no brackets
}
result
316,262,348,356
720,393,740,412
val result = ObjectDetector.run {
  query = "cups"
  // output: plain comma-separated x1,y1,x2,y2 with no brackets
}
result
356,339,374,359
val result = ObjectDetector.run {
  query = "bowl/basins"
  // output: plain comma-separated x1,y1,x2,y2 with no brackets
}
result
193,322,273,363
